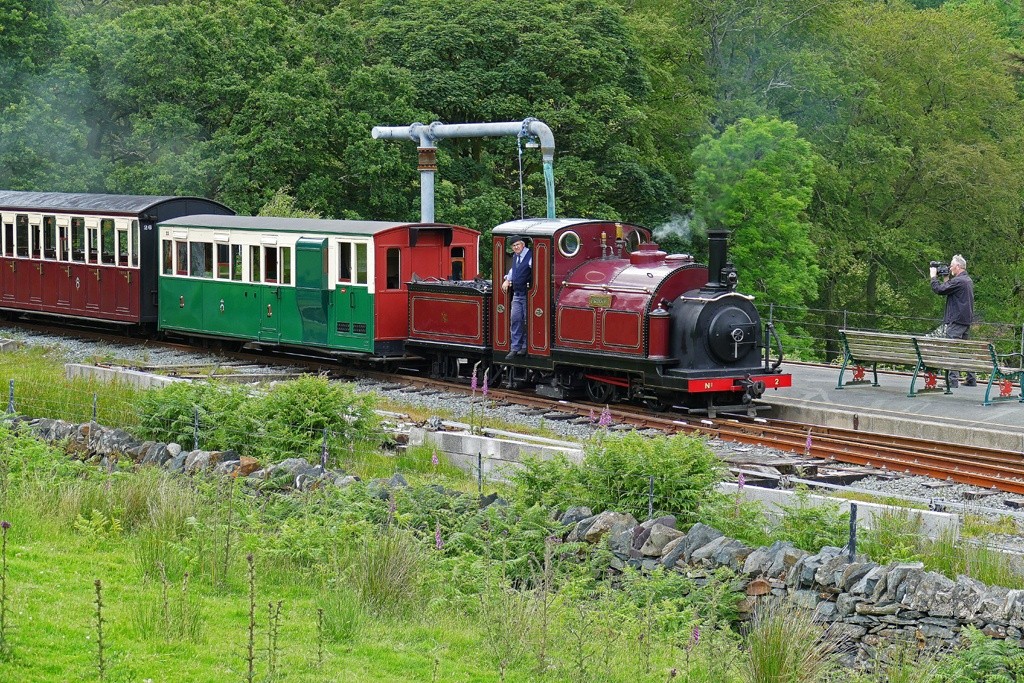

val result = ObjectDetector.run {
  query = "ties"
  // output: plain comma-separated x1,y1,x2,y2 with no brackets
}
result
518,255,520,264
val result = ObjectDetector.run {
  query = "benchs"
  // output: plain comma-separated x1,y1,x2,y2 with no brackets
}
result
835,329,1024,406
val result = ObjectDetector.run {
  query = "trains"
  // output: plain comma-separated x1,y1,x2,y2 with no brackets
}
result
2,116,791,416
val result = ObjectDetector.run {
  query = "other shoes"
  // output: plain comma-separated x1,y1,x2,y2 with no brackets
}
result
505,351,517,359
517,348,527,355
938,383,958,388
961,382,976,386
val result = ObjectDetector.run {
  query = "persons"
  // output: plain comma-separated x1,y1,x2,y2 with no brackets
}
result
929,255,977,388
500,234,533,360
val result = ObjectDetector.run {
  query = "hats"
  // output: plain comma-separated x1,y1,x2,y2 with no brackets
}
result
509,235,522,245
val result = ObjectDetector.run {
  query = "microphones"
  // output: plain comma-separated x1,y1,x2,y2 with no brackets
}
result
930,261,948,266
